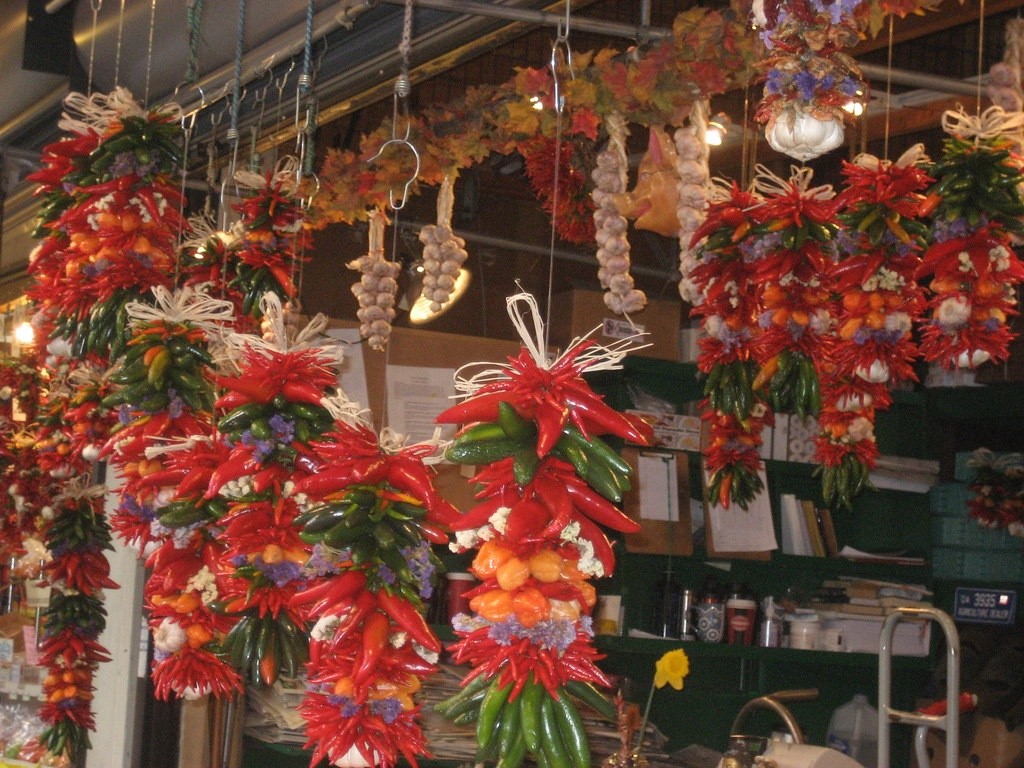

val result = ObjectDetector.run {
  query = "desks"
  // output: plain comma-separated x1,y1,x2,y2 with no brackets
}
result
589,635,928,768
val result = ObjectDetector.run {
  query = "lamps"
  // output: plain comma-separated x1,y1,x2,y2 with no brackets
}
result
396,250,473,327
838,81,869,116
703,113,731,146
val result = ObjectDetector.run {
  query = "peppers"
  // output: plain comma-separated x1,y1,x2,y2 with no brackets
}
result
0,114,339,768
689,134,1024,534
286,335,655,768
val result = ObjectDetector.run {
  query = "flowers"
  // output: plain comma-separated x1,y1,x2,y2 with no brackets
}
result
636,647,690,750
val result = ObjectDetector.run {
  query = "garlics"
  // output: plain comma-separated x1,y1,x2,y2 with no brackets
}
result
182,681,213,701
590,63,1021,435
262,226,469,351
82,445,108,461
153,617,188,652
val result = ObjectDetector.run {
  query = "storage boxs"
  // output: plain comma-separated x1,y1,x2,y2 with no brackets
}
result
927,483,1024,582
909,695,1024,768
547,290,683,363
621,408,700,452
974,317,1024,386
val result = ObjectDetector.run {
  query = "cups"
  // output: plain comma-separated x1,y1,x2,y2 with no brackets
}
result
697,600,727,644
725,597,756,646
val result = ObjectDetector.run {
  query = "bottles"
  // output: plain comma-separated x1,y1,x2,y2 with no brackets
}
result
825,694,881,768
677,588,699,642
757,602,783,648
788,621,823,650
